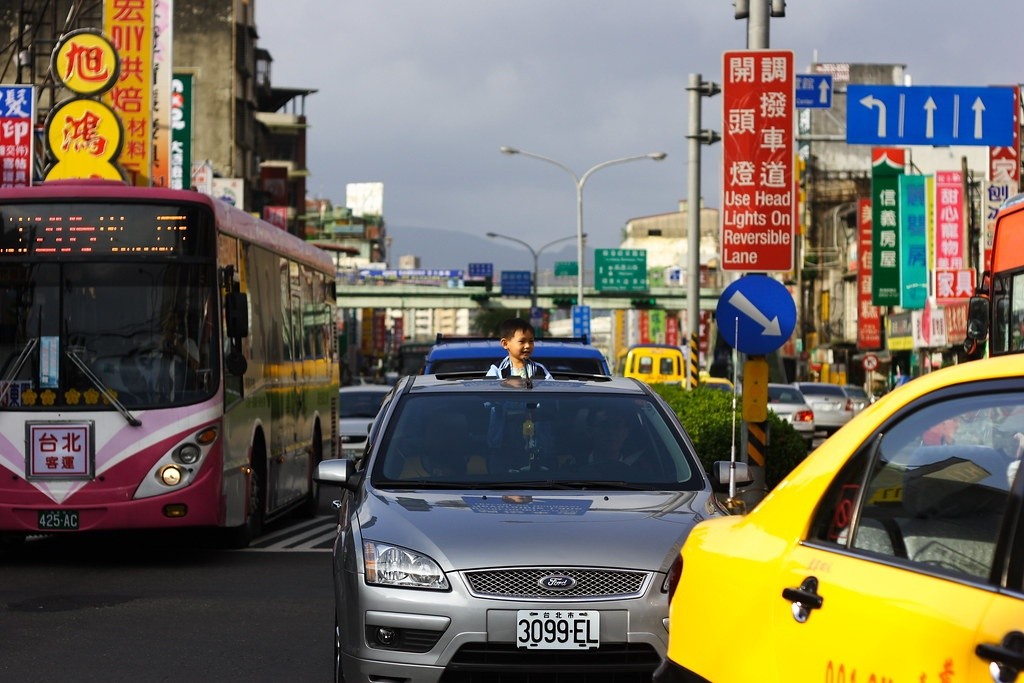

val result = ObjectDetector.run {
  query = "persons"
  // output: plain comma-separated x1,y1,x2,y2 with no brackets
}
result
481,317,557,449
566,403,642,484
129,278,200,371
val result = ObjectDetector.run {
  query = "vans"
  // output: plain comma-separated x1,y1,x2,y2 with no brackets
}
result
627,345,684,388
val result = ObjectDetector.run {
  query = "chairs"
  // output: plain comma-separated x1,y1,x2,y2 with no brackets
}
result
835,445,1012,592
779,392,793,401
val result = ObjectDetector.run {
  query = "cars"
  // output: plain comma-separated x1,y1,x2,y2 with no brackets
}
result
763,383,815,449
315,373,753,683
665,353,1024,682
793,381,854,440
697,370,735,391
338,383,393,459
841,384,870,415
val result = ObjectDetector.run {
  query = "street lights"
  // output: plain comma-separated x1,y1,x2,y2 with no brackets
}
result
485,231,588,309
497,144,669,305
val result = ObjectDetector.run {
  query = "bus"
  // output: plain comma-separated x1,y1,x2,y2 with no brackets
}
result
390,341,434,379
0,175,339,552
962,201,1024,360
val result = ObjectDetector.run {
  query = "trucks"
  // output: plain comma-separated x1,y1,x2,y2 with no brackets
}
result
424,332,613,379
